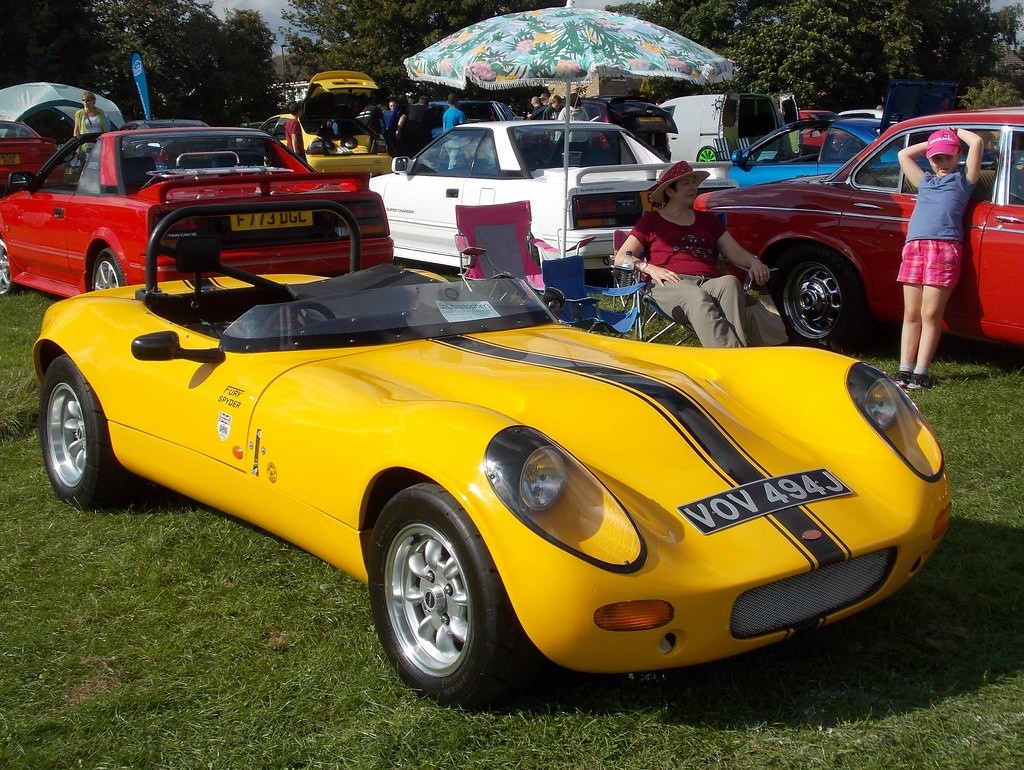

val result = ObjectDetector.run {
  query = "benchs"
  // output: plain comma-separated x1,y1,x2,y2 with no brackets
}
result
898,169,997,197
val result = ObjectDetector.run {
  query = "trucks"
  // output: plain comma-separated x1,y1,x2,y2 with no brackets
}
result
660,94,782,162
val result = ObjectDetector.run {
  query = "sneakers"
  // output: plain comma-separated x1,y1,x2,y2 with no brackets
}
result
907,373,929,390
894,369,910,388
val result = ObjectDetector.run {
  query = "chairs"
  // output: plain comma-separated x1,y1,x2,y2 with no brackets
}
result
102,155,157,193
454,200,780,346
832,138,856,160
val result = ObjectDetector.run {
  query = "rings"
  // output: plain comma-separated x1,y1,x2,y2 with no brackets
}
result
763,271,767,274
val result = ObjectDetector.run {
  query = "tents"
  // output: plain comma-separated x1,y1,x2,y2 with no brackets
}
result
0,82,125,137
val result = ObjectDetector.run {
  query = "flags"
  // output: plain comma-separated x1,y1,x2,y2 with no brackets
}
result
132,53,150,120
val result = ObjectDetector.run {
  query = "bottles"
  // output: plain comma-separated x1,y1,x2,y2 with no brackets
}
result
620,251,634,287
258,168,272,195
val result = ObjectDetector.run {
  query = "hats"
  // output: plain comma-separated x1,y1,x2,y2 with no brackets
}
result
924,130,960,159
650,160,710,203
539,94,549,99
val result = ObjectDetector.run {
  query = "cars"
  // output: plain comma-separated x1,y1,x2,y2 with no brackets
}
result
526,98,679,171
369,120,738,273
258,71,392,178
728,81,1014,192
0,120,393,297
389,102,523,159
693,106,1024,355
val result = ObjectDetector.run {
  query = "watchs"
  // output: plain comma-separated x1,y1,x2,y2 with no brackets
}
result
642,262,649,271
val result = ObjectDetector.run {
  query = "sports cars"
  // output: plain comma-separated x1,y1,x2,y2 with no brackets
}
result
33,199,951,713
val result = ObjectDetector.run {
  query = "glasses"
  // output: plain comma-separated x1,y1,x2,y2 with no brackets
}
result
82,99,92,102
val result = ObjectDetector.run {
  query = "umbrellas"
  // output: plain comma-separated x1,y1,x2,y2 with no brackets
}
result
404,0,734,259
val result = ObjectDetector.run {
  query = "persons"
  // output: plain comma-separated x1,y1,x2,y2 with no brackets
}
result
614,161,771,349
527,93,592,157
73,92,107,166
285,103,307,162
894,128,984,394
356,96,436,158
442,93,472,170
